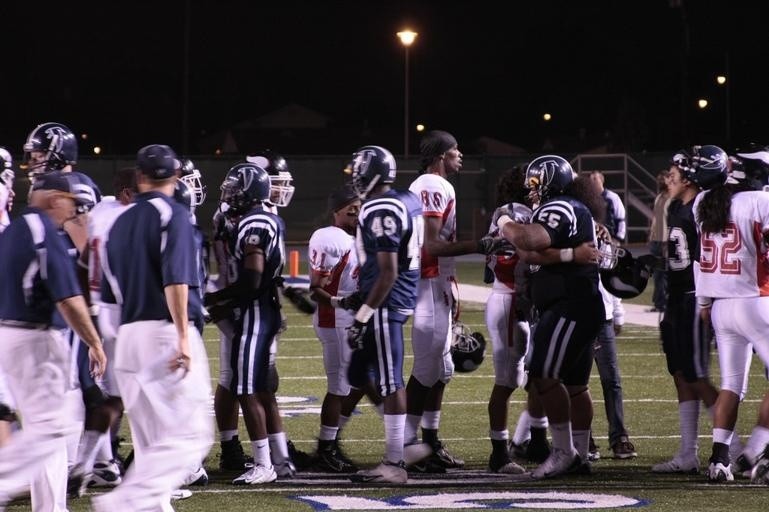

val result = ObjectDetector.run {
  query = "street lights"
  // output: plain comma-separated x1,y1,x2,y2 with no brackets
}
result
397,27,419,183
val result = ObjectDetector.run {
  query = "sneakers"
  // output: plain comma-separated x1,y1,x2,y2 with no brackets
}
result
219,435,255,476
750,443,769,486
315,437,359,474
232,463,277,486
405,460,448,474
430,444,467,469
733,453,753,479
651,456,701,476
587,438,601,461
286,439,310,469
704,457,734,482
507,441,530,462
78,458,122,497
489,453,527,475
273,458,297,479
377,459,408,484
168,487,193,501
188,463,210,487
528,447,585,480
610,435,638,459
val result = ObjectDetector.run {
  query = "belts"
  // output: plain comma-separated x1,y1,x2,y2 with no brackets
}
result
0,318,58,331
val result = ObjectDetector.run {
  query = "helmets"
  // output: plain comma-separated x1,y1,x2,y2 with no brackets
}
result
244,148,296,208
175,159,208,207
595,241,652,300
218,161,275,212
687,145,729,190
450,331,486,373
23,121,79,180
350,145,398,200
65,172,104,216
523,154,574,212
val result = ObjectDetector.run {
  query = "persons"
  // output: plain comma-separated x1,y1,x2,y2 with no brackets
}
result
21,125,119,510
92,146,218,511
219,164,295,485
654,155,746,475
403,131,516,473
215,156,312,470
650,174,674,312
82,166,134,458
0,150,16,455
566,175,638,459
696,152,767,484
168,158,210,350
587,170,628,334
311,187,368,472
342,147,425,482
0,171,108,511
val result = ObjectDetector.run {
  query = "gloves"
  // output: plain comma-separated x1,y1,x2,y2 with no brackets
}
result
344,319,367,351
337,291,362,313
281,286,319,315
478,226,516,258
491,203,516,226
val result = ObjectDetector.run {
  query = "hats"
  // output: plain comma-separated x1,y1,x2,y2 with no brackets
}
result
31,171,79,201
134,143,181,183
